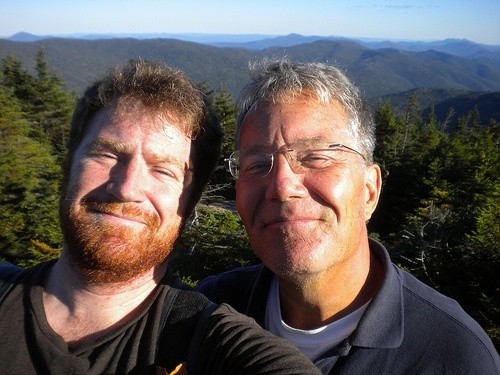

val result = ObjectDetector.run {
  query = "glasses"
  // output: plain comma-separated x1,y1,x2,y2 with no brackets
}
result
223,145,367,180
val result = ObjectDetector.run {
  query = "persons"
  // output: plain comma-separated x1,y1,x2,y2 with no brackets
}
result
189,59,500,375
0,58,323,375
